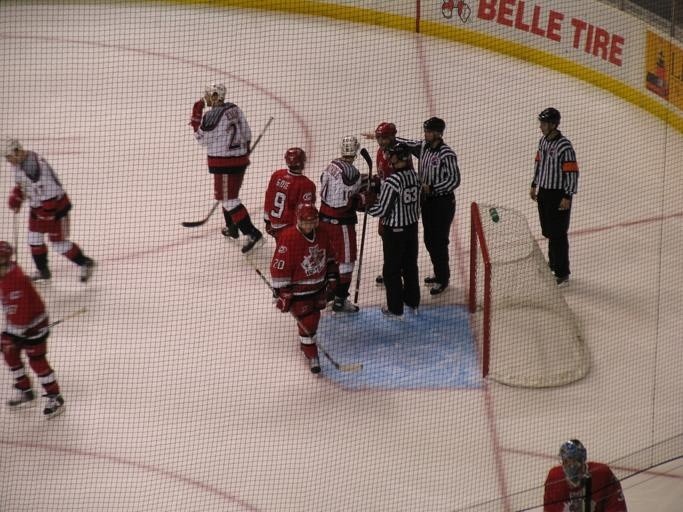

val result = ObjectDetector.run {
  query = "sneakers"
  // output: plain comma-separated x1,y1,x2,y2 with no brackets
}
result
552,274,568,285
332,300,359,313
30,270,49,280
376,275,449,316
221,227,238,238
9,388,33,405
308,357,321,372
80,257,92,281
242,228,261,253
42,393,63,414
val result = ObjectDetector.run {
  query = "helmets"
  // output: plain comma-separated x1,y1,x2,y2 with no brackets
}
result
0,240,12,265
0,138,18,156
204,82,224,101
284,148,305,167
295,203,319,229
339,117,444,160
558,439,585,475
538,107,559,124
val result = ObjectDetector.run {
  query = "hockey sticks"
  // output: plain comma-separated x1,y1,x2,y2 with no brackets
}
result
354,148,372,303
181,116,273,227
255,268,363,373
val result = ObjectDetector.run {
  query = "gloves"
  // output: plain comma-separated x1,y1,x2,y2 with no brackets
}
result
191,101,203,126
36,210,55,230
8,188,23,207
0,334,15,356
275,288,292,312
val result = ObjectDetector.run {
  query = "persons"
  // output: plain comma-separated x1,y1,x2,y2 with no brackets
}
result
189,82,262,254
0,239,64,416
543,439,628,511
369,121,413,284
0,138,94,283
264,147,316,240
361,141,422,319
271,203,341,375
317,134,376,313
360,116,461,295
529,107,579,287
